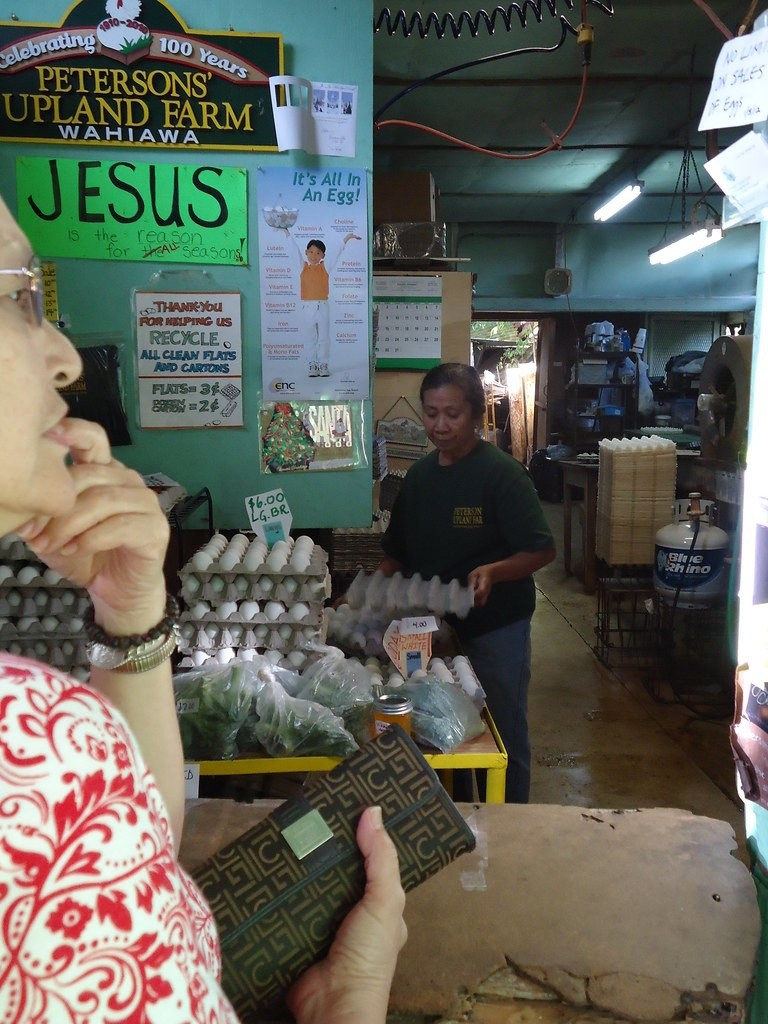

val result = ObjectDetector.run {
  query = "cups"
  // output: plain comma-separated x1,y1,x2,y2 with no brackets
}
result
373,693,413,742
632,328,647,354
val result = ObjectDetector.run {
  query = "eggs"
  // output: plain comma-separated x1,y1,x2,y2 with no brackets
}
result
0,532,479,697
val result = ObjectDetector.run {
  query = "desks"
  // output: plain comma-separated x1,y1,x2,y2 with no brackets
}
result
559,460,598,594
185,706,510,805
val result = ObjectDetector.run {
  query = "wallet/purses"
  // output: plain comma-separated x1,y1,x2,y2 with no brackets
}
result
186,722,477,1023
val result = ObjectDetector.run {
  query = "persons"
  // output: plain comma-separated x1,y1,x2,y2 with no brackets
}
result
275,227,362,377
333,363,558,803
0,197,408,1024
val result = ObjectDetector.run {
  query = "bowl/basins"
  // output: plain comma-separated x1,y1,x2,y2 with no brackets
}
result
262,208,300,229
655,415,672,426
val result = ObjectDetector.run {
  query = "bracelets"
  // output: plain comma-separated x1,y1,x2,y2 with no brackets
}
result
81,593,181,674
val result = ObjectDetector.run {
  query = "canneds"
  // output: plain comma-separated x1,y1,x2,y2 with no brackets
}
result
373,694,413,741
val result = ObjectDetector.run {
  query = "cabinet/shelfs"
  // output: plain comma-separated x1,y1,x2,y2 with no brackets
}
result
561,308,646,456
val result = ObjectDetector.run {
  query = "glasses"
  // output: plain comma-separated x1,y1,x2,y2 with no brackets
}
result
0,255,44,328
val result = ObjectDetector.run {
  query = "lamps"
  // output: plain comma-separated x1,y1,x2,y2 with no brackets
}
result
592,178,644,221
648,219,729,265
